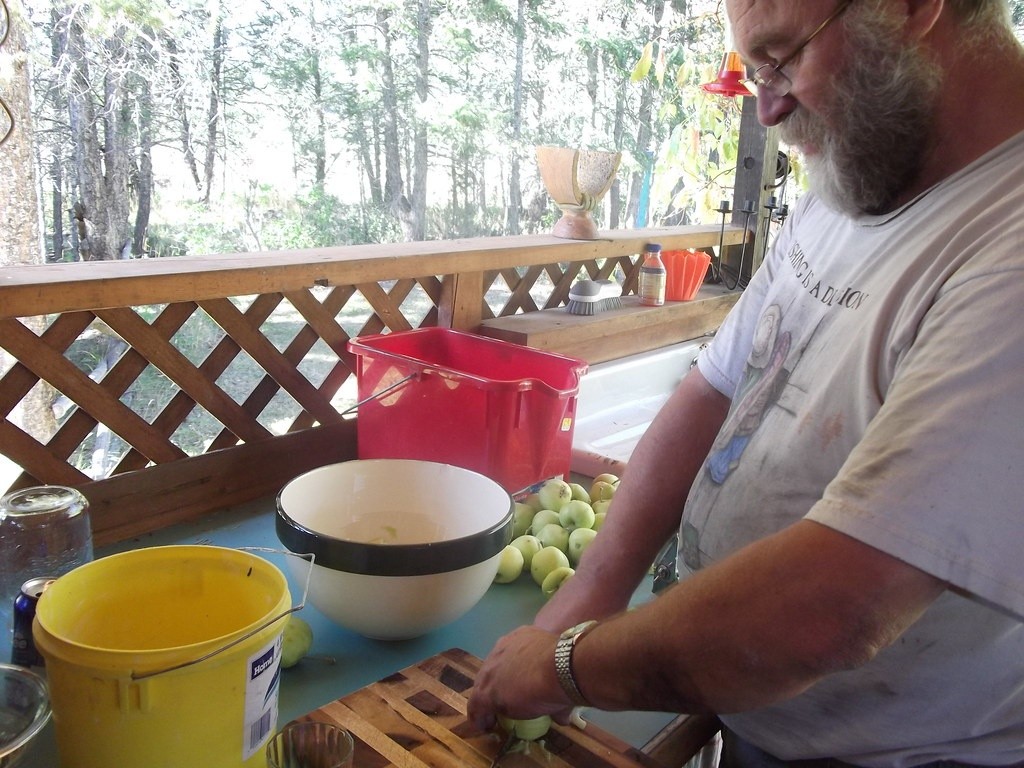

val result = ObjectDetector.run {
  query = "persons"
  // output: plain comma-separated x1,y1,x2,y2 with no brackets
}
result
466,0,1024,768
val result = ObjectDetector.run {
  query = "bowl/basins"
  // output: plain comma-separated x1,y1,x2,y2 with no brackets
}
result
0,663,52,758
276,458,515,641
661,251,711,301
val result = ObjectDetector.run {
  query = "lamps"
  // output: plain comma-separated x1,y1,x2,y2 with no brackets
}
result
703,52,753,99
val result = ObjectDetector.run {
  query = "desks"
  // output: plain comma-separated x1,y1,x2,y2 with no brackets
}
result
0,456,721,766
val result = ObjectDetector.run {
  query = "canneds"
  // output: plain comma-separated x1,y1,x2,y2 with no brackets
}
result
10,577,60,707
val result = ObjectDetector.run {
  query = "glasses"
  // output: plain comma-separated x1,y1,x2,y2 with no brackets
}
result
738,0,851,97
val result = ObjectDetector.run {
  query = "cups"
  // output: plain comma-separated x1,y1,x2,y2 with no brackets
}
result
1,487,95,675
267,721,353,768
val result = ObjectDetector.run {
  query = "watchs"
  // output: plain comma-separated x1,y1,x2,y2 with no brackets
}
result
554,620,598,706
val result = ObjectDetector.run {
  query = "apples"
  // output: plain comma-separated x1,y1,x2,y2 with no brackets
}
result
494,711,551,740
495,474,621,599
281,619,313,669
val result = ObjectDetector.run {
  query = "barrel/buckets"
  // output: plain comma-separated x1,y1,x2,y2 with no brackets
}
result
33,543,291,767
348,326,590,492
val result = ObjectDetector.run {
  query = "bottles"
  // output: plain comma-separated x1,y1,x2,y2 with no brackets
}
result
640,242,667,306
